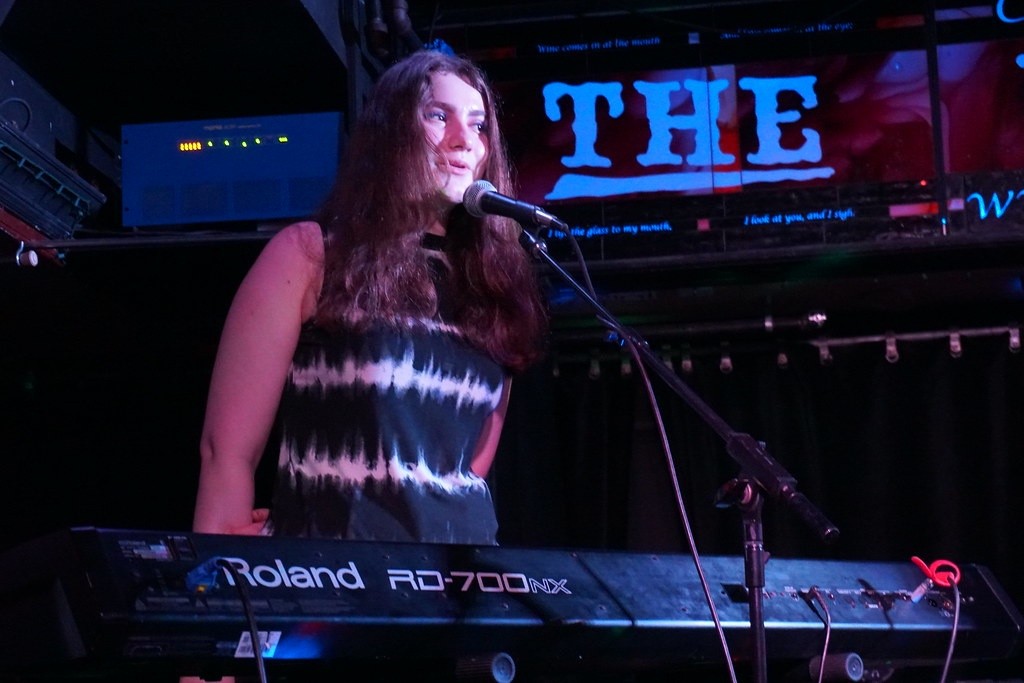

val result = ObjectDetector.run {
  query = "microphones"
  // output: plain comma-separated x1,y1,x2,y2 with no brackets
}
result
463,179,569,232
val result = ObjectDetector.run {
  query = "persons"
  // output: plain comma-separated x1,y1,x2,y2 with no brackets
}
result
182,52,552,683
